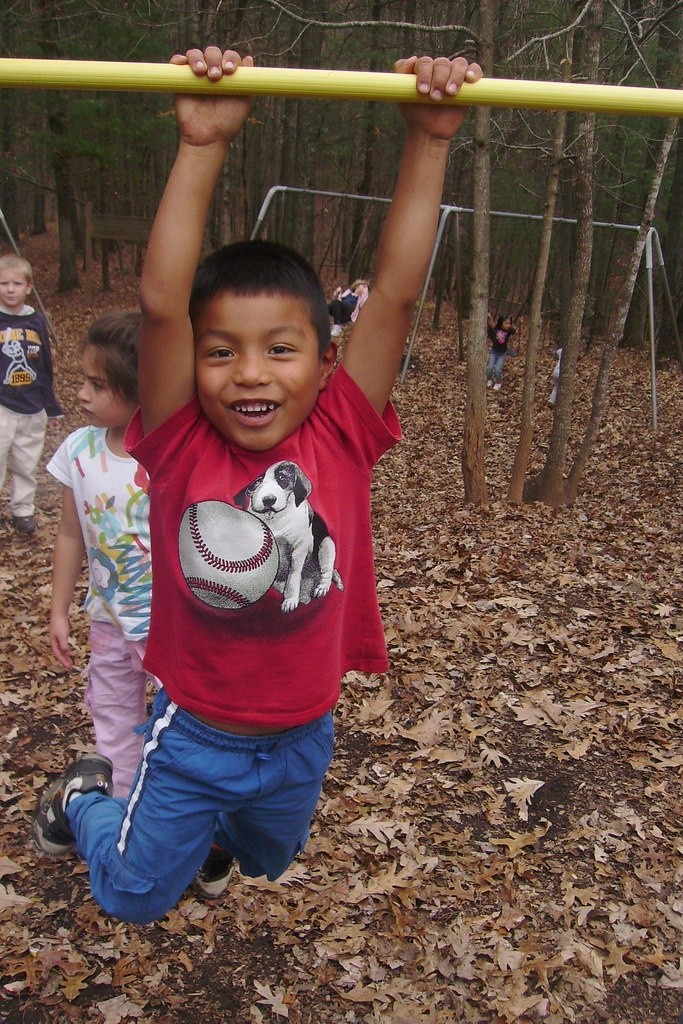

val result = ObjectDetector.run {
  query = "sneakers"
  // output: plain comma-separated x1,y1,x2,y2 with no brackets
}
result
32,754,112,855
192,847,234,898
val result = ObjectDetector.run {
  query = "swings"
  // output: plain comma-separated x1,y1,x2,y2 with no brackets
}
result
552,221,620,366
314,196,374,314
483,215,546,336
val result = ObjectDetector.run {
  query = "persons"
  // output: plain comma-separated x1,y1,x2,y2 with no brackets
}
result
34,45,484,924
329,278,370,340
488,312,524,390
0,254,66,535
49,310,235,898
547,341,591,410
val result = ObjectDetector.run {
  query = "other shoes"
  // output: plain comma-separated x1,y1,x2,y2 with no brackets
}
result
330,324,342,337
486,380,492,387
12,515,34,533
492,383,501,391
548,399,554,408
